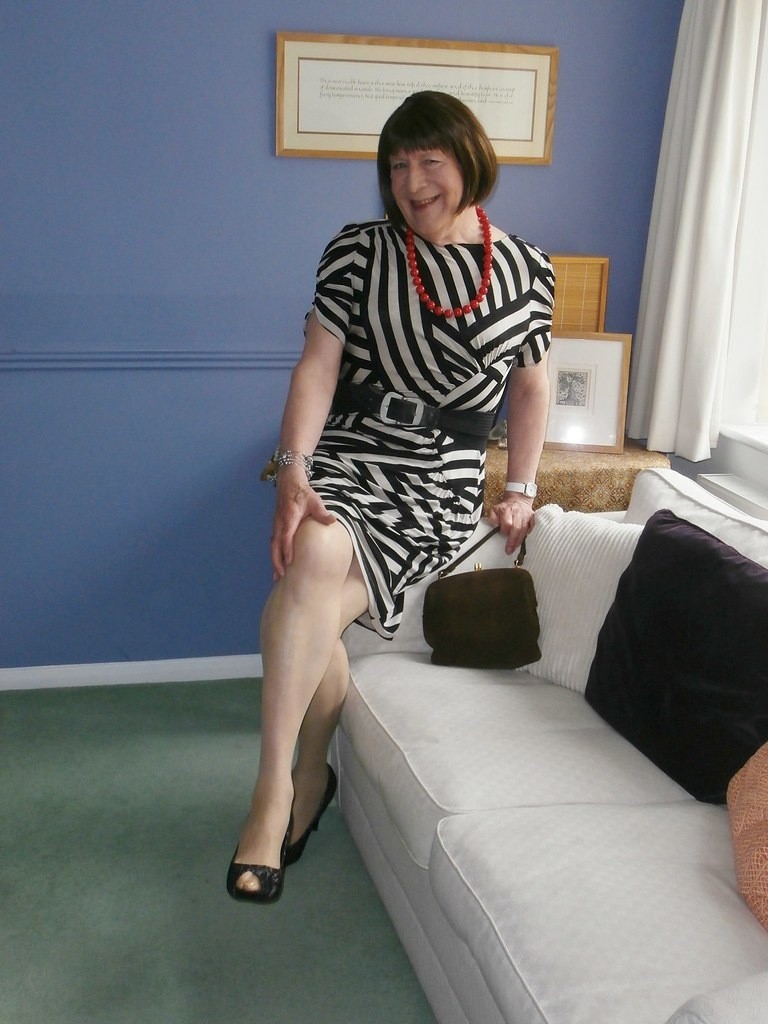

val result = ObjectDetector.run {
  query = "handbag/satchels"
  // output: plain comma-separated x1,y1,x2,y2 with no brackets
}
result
423,527,541,670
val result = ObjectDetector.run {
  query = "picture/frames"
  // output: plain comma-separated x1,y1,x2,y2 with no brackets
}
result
496,330,632,455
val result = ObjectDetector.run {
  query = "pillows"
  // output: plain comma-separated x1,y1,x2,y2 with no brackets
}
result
583,509,768,803
726,739,768,929
505,503,645,694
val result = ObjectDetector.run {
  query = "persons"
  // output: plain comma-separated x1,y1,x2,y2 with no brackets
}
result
225,90,555,907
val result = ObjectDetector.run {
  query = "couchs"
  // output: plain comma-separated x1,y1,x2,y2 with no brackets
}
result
325,469,768,1023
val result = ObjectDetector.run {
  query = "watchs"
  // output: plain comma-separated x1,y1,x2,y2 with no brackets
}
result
504,481,537,499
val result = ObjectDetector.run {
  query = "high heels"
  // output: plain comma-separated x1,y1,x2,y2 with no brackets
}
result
226,816,295,900
285,764,337,865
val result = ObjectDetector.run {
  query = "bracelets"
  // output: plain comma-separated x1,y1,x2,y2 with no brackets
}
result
259,450,315,484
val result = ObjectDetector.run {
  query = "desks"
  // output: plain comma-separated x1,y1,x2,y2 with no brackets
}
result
481,442,671,519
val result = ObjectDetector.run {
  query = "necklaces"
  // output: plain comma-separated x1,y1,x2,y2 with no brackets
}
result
405,204,493,319
